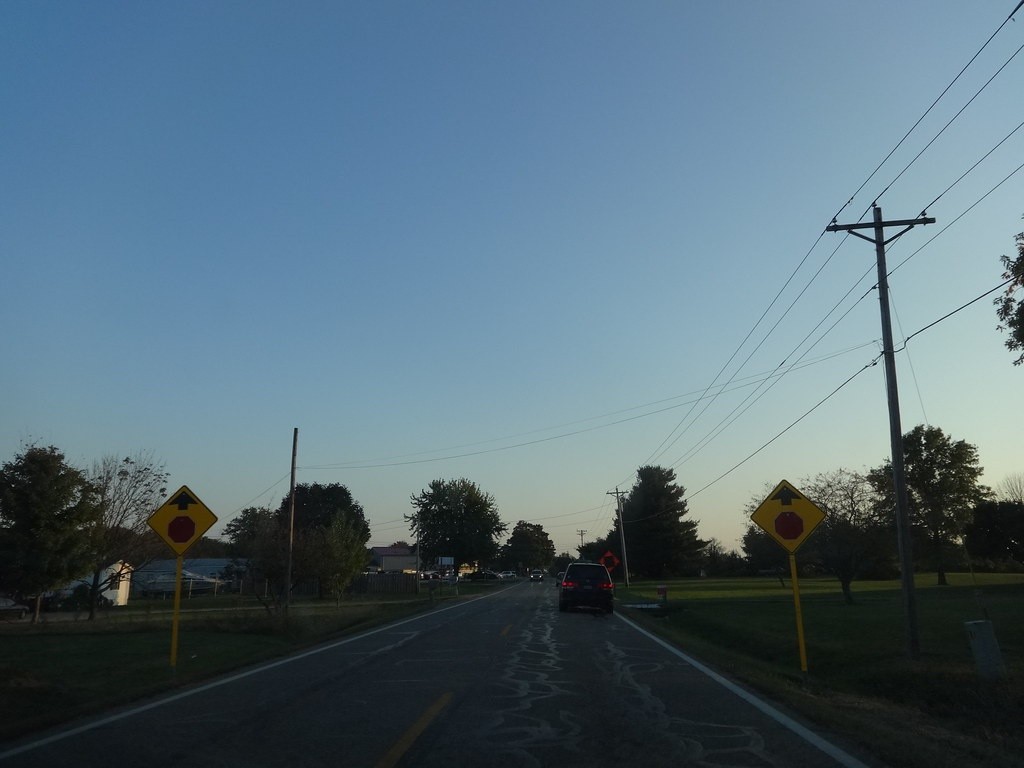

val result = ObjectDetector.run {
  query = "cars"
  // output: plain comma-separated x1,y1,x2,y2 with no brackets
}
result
466,570,498,580
529,569,544,582
555,571,566,587
496,571,519,584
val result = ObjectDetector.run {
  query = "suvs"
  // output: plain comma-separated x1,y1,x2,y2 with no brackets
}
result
557,561,615,615
